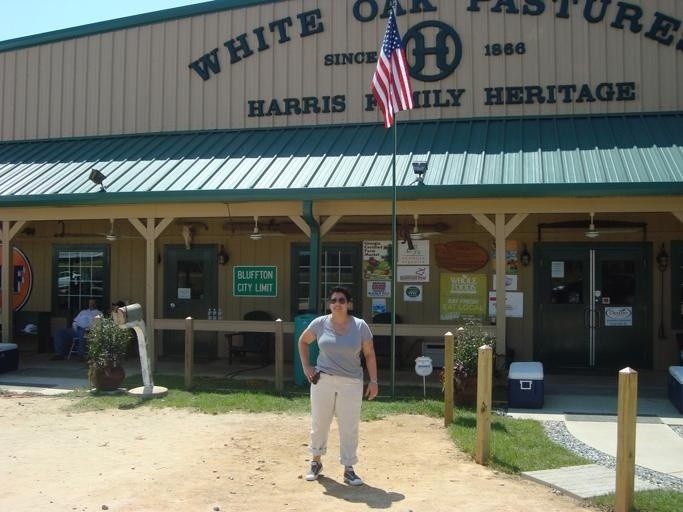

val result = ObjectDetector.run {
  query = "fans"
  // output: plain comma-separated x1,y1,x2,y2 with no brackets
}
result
249,216,288,241
584,213,643,239
96,219,144,241
408,215,443,240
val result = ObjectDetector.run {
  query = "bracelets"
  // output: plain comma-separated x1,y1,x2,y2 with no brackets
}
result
367,379,380,385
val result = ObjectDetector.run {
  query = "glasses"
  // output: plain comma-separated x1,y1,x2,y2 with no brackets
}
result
328,298,345,304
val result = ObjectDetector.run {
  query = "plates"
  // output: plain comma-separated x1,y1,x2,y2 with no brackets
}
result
366,270,391,277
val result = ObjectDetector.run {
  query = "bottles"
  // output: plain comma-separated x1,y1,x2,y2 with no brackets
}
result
217,308,223,320
212,309,216,320
207,308,211,320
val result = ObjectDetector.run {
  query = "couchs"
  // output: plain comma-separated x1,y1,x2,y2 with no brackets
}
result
0,311,50,353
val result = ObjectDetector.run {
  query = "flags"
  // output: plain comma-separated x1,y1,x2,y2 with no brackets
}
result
370,6,415,129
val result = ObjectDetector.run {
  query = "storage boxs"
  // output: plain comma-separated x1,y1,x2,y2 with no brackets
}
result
0,343,19,374
508,362,544,408
667,365,683,415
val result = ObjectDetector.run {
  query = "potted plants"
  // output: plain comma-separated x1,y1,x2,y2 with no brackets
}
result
439,320,497,407
83,314,134,391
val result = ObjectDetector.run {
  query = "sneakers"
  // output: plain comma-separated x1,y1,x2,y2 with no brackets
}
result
344,466,362,485
306,461,322,480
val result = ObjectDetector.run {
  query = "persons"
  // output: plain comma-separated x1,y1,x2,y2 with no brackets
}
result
298,286,379,486
111,300,126,321
48,296,106,363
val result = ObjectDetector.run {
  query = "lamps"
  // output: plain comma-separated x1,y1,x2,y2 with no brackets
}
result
520,245,530,269
656,242,668,272
217,244,229,266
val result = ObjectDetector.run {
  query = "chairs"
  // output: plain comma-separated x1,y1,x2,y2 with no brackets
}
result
224,311,275,365
361,312,401,369
47,318,67,355
67,334,90,361
